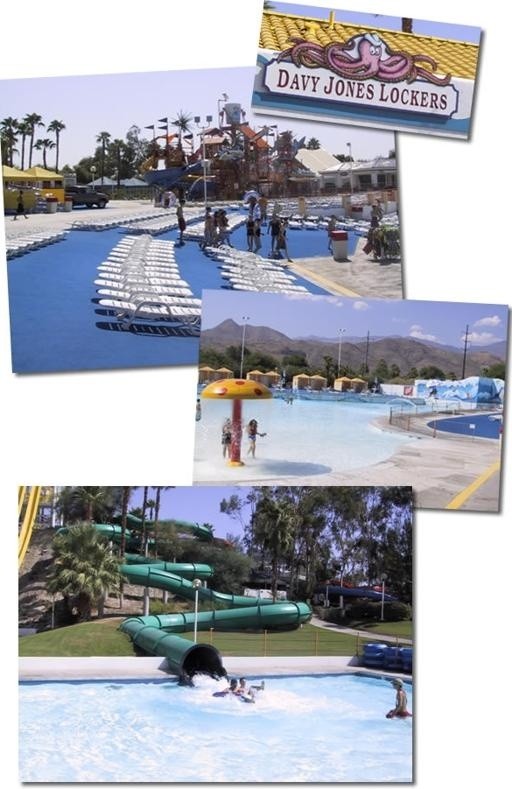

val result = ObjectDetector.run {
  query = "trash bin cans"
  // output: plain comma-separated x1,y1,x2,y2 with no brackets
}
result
352,206,363,220
331,231,348,261
47,198,58,214
64,196,74,212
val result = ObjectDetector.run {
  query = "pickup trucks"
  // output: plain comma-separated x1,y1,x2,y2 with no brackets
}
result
65,185,109,208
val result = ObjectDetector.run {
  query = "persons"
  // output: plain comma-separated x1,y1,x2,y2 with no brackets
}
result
244,419,266,457
156,187,386,264
13,190,28,220
221,417,233,459
238,677,265,703
224,677,247,702
386,678,413,718
196,398,201,422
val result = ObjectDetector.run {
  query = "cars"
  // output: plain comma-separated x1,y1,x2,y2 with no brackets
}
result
330,578,353,589
265,563,292,575
368,582,394,593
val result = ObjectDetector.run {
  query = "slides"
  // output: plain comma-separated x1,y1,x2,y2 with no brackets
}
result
54,513,313,687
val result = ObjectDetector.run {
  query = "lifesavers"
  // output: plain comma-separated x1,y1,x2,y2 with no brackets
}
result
363,643,413,672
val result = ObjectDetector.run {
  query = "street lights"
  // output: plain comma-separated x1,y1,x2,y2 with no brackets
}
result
347,142,353,195
338,328,346,378
194,115,213,213
111,175,115,194
323,579,330,608
192,578,202,643
379,573,387,621
240,316,250,379
90,166,97,190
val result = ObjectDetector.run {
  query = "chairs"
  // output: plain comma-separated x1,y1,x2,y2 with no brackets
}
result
4,190,400,337
